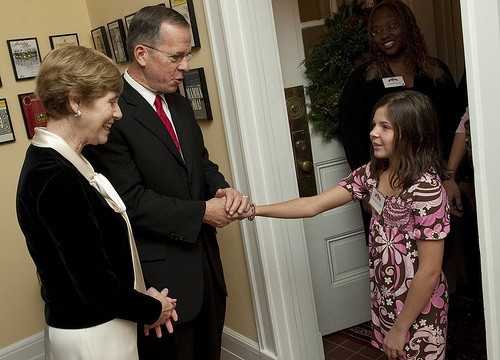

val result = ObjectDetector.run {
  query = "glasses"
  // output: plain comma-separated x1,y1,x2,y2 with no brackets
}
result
370,24,401,36
141,43,193,62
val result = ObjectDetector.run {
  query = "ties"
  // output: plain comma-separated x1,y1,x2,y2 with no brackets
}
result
155,94,183,159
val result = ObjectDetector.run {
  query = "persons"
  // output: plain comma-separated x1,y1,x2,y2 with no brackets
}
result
12,46,40,68
441,105,470,217
338,0,468,360
87,5,252,360
96,35,105,57
16,41,178,360
113,29,124,62
227,89,452,360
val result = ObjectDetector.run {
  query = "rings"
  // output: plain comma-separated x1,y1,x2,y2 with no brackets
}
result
241,195,249,199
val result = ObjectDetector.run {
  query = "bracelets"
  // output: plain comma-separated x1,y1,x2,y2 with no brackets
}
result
248,203,257,222
443,168,456,180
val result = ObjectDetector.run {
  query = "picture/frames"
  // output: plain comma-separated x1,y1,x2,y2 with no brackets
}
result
0,0,213,145
